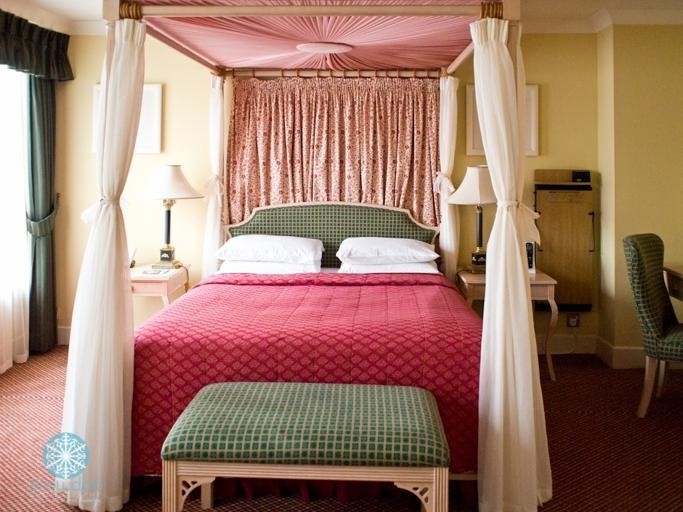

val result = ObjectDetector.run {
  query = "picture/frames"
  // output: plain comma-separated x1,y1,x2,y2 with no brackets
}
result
92,83,162,154
465,81,539,157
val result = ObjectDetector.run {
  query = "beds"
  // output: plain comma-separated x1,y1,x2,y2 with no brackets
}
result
130,201,483,482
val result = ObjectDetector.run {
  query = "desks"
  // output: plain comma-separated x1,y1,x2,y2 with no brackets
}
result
128,264,189,306
664,261,682,302
458,265,558,381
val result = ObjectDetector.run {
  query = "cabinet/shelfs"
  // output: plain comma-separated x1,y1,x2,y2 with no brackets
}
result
533,185,595,311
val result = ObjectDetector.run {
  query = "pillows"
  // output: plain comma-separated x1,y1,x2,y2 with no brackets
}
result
336,237,440,265
216,261,322,274
337,262,442,274
216,234,326,266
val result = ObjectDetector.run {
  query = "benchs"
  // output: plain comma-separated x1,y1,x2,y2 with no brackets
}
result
160,381,451,511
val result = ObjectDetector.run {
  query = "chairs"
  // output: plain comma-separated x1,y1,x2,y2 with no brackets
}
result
623,233,683,419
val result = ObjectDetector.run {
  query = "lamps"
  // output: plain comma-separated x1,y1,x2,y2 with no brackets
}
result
140,164,205,268
444,164,496,273
